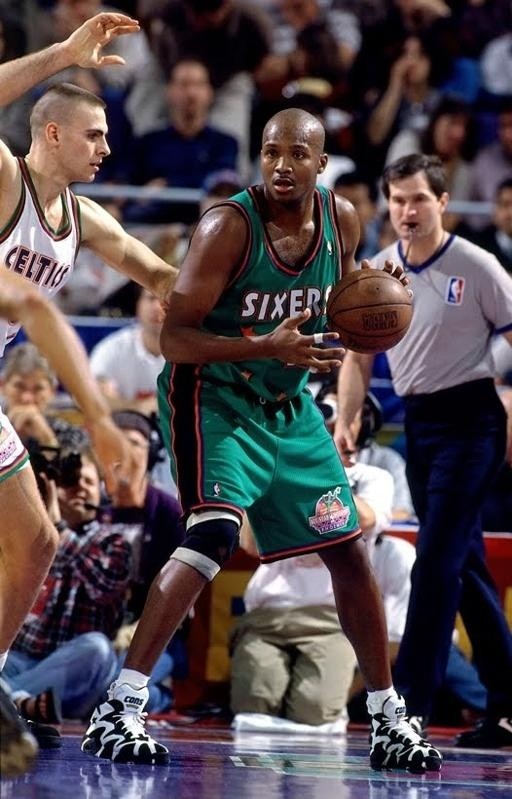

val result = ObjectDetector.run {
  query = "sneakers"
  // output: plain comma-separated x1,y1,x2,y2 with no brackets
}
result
0,680,64,779
81,683,170,761
367,696,442,771
454,718,512,748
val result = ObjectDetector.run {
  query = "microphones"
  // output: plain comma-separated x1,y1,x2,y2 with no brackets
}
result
84,503,105,512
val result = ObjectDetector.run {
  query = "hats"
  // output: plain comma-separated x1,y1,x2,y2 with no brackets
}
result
203,170,243,197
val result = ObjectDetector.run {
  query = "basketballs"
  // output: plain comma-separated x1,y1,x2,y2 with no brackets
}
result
328,270,414,352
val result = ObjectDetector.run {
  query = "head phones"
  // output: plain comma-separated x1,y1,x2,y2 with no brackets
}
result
356,389,385,449
113,409,166,471
100,477,113,506
